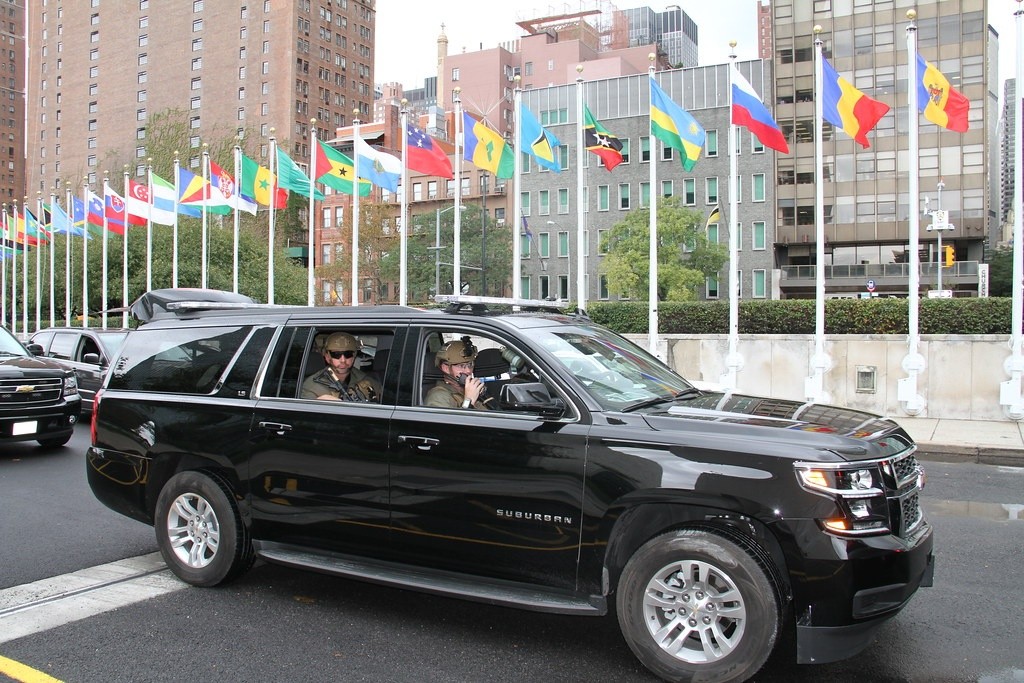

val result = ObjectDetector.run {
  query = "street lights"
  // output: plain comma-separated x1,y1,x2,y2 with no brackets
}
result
926,179,955,299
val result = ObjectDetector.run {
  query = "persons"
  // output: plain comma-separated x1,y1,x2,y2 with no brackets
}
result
423,341,491,410
300,332,384,401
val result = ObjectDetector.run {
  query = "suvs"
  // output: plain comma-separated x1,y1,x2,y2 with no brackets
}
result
84,286,938,683
26,326,134,417
0,323,83,448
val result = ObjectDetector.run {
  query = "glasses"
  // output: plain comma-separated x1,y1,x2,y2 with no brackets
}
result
329,350,355,359
453,362,474,370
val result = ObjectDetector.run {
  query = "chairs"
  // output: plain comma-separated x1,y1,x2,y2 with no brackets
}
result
305,352,326,378
473,348,510,401
422,352,445,401
366,349,390,384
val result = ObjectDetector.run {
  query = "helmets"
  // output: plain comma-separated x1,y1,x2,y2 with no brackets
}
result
324,331,359,351
434,341,475,367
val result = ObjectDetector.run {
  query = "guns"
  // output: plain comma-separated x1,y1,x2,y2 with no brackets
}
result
314,365,369,404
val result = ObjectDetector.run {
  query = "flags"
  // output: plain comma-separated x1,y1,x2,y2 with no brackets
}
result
650,75,706,172
583,106,624,172
916,53,969,132
275,146,326,201
520,105,562,172
462,111,514,178
316,138,372,195
0,173,175,260
816,54,891,149
358,133,401,194
729,65,790,154
210,160,260,217
241,153,286,211
178,167,231,215
